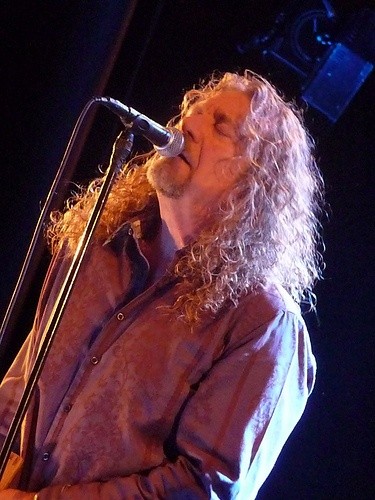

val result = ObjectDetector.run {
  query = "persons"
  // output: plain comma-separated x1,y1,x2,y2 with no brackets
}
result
1,67,327,500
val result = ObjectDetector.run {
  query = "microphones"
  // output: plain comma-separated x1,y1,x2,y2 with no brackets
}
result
90,95,186,159
